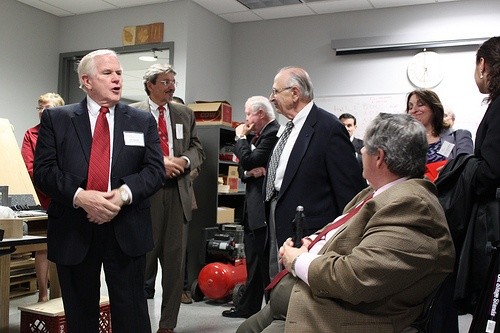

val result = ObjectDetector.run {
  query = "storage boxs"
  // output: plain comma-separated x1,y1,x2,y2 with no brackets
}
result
187,101,232,126
17,295,112,333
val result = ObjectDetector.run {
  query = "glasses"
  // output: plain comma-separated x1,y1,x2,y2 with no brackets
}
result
154,78,176,86
35,107,49,112
272,86,294,97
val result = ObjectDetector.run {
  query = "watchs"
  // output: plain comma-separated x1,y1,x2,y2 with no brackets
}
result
235,135,244,141
118,188,129,205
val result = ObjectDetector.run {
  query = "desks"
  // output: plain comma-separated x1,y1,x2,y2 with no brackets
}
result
0,214,62,333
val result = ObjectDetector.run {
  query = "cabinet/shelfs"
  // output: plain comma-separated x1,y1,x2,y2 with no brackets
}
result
186,124,256,292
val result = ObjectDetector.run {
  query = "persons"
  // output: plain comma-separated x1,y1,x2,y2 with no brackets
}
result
337,113,364,173
138,97,192,305
222,95,283,319
261,67,369,280
33,50,167,333
436,36,500,333
441,109,475,155
20,93,65,304
126,64,204,333
239,113,456,333
406,89,455,183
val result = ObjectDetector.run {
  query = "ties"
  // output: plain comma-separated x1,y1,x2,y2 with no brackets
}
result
265,195,372,290
158,106,170,156
251,132,261,146
265,120,294,202
86,106,109,192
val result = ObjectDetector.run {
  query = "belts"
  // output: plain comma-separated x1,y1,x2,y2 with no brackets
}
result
272,191,279,197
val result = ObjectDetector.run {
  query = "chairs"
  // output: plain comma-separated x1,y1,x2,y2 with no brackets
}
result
411,153,500,333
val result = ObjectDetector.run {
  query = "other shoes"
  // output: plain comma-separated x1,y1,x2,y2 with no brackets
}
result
180,291,192,304
222,308,251,319
157,326,174,333
